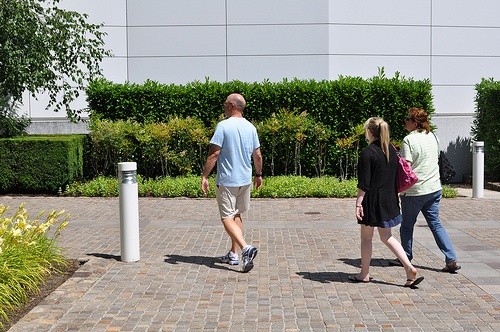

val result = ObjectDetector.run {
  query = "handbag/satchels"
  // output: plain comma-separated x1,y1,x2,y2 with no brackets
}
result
390,143,418,192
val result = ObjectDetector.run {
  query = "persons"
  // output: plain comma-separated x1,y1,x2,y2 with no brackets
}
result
391,108,461,272
348,117,424,287
201,93,262,272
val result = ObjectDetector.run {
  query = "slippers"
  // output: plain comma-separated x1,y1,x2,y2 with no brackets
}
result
442,263,461,272
348,274,370,283
404,276,424,287
388,258,414,266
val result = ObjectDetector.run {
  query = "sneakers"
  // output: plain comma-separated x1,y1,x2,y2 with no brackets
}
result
242,246,258,273
218,250,239,265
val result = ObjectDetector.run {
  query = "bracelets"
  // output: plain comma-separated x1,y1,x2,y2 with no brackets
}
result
356,205,362,207
255,173,262,177
